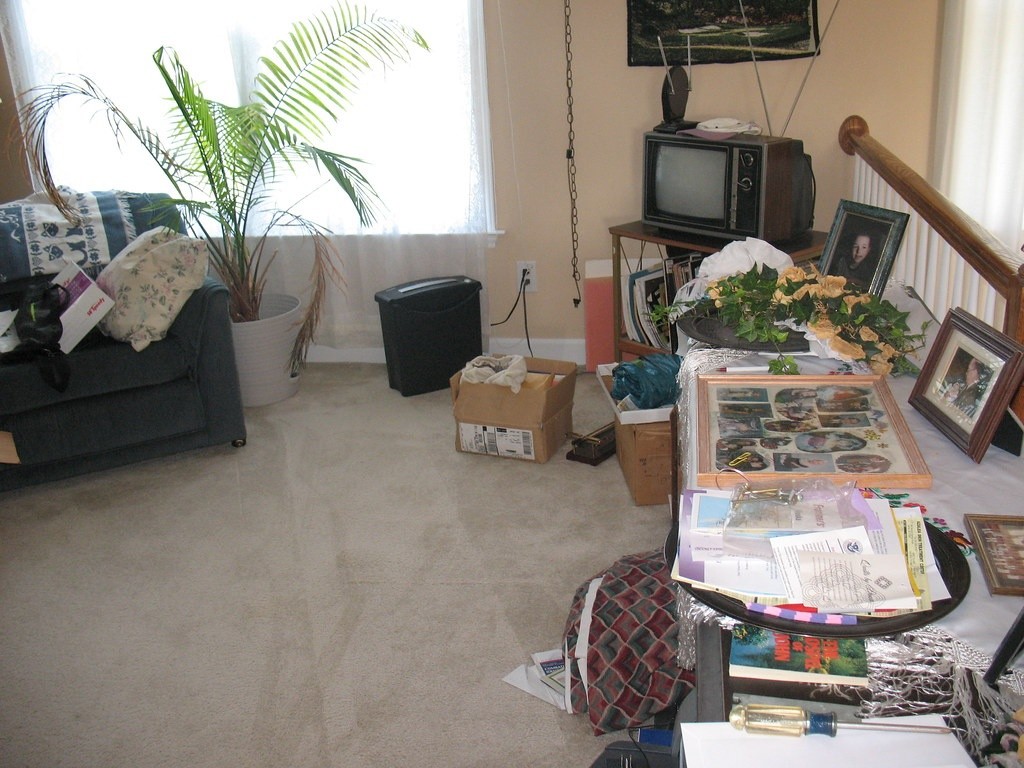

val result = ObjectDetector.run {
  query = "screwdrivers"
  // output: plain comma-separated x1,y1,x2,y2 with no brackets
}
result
729,703,952,738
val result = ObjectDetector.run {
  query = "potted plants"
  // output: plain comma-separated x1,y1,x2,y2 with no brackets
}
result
0,0,432,408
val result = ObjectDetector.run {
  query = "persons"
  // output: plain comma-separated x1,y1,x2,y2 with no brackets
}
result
830,228,879,293
715,385,887,474
942,357,984,419
982,528,1024,580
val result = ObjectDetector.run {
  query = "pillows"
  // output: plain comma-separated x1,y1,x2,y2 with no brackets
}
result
94,225,210,353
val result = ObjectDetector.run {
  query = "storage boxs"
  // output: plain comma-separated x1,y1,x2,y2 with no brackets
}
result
595,363,676,425
615,414,672,506
449,354,578,464
51,260,116,355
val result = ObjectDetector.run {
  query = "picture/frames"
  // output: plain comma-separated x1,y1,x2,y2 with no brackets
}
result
816,199,910,302
964,514,1024,596
908,307,1023,464
696,374,932,490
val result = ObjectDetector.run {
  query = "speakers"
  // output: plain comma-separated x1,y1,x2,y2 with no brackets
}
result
653,34,700,134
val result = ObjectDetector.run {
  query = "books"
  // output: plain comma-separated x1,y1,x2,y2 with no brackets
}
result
621,251,702,356
52,260,115,354
671,489,953,687
595,363,675,425
530,650,565,696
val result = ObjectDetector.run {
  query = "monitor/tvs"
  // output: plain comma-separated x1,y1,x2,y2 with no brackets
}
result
642,0,841,247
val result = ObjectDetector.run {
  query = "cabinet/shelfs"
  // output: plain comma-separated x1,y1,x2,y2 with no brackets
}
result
608,220,828,362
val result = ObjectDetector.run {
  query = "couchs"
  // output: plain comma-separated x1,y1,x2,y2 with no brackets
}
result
0,184,246,494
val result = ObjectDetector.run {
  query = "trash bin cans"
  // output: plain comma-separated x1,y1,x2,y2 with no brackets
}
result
375,275,482,397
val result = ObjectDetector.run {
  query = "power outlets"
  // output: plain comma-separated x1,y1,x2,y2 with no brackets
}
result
517,260,536,292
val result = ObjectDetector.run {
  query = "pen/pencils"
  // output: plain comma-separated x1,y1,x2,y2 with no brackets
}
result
715,365,789,372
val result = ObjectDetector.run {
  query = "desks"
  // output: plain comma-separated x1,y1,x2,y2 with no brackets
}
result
676,286,1024,724
680,712,980,768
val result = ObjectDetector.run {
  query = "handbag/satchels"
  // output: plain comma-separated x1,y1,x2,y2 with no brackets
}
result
0,281,71,392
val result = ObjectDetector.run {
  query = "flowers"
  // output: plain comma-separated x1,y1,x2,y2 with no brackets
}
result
641,260,934,378
